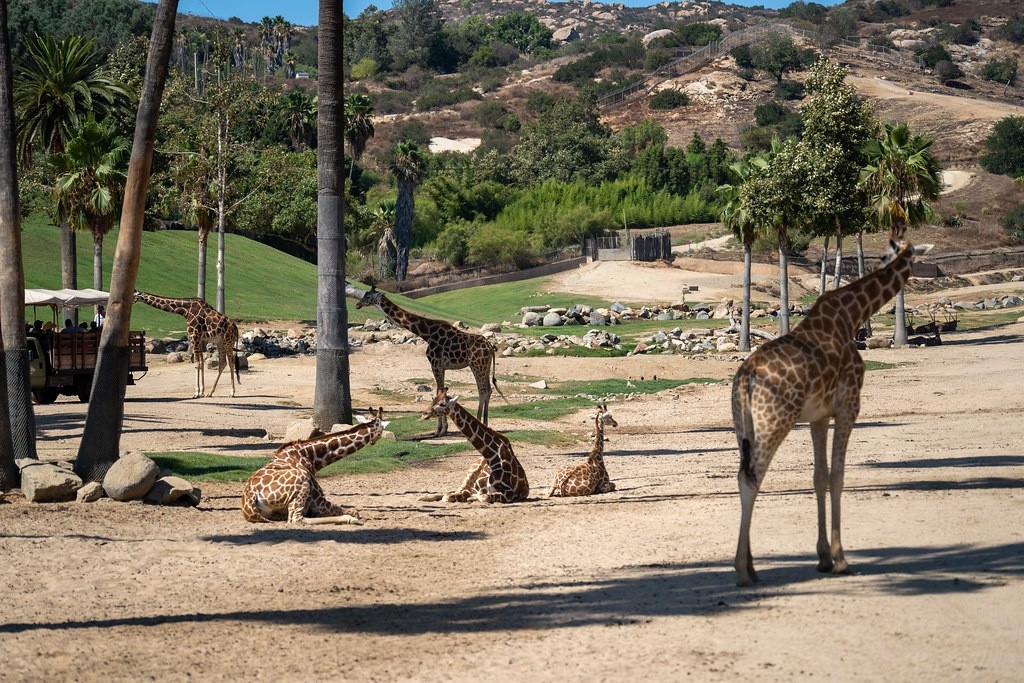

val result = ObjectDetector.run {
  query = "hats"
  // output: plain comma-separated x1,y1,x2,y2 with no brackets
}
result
34,320,44,326
44,322,56,329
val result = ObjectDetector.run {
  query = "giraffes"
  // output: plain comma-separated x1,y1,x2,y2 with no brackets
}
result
241,406,383,526
417,386,530,505
548,403,619,497
132,289,242,398
732,235,936,587
355,286,512,439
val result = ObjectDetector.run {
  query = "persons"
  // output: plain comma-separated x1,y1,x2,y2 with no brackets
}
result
61,319,78,334
43,321,56,333
25,323,30,333
78,322,88,332
89,322,98,333
94,305,106,327
33,320,44,333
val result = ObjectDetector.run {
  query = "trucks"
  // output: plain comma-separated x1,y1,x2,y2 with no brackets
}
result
23,287,148,403
